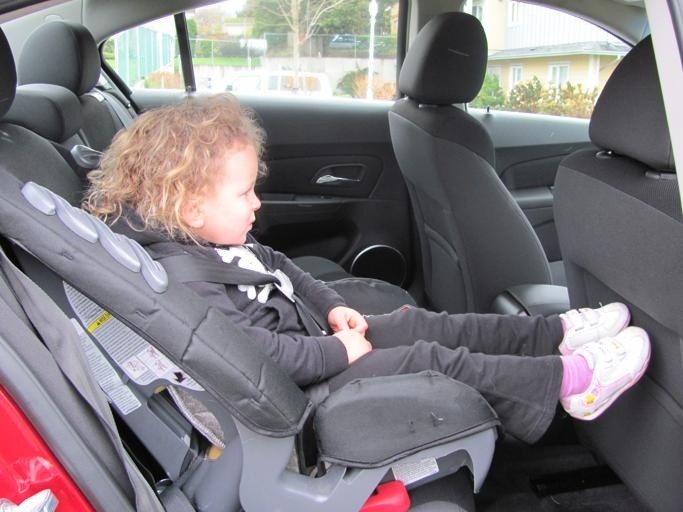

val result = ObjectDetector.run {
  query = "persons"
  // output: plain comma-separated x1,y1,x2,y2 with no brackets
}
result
74,88,654,469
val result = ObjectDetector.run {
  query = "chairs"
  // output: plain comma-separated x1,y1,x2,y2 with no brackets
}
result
552,29,683,512
385,11,572,317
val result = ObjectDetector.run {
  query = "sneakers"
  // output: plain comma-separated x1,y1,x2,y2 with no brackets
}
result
558,301,632,357
560,326,653,422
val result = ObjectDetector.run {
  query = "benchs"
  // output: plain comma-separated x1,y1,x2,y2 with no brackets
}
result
1,20,502,512
